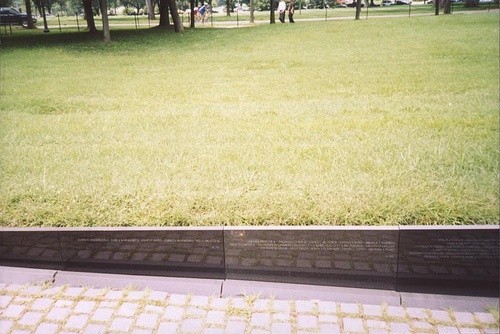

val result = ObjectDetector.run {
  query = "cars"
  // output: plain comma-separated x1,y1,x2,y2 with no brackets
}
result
0,7,37,27
106,1,406,16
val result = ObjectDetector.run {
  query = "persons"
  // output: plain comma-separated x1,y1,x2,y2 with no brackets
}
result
199,2,208,23
193,4,201,22
288,1,295,23
277,0,287,23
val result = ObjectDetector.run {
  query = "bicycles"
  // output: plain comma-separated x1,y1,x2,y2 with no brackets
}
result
196,12,215,25
